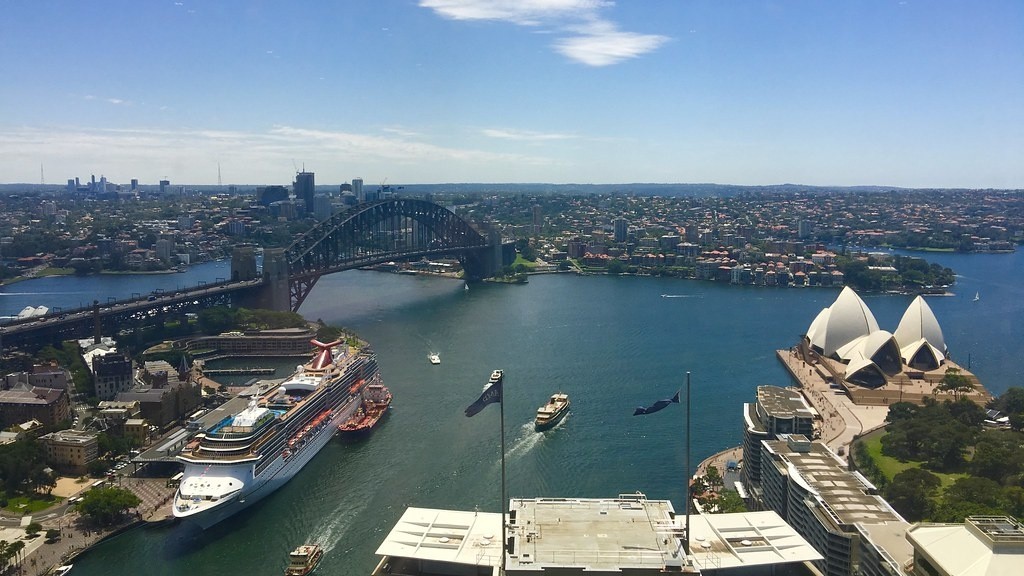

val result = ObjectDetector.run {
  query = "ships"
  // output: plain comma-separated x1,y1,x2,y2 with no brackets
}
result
171,336,385,531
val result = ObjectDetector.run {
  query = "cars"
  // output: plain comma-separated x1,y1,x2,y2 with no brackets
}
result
104,458,132,477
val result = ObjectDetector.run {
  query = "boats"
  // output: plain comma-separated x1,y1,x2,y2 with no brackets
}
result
334,373,393,436
282,408,333,460
429,351,442,364
534,391,571,429
483,370,505,392
284,544,325,576
351,377,367,394
53,563,74,576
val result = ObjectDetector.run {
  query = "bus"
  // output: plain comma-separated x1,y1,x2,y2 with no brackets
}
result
68,496,83,504
92,480,104,490
190,409,204,423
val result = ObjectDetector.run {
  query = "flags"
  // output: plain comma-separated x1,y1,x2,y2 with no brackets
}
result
464,379,501,417
632,391,680,416
